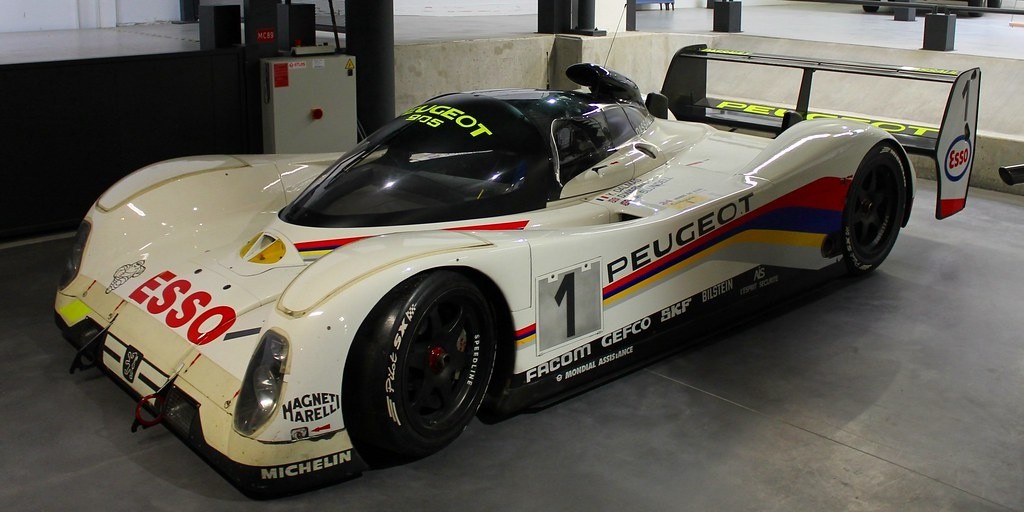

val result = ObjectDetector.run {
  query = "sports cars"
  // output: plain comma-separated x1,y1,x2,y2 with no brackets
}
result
52,45,983,500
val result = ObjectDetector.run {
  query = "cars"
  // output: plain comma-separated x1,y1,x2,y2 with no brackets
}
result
863,0,1003,18
